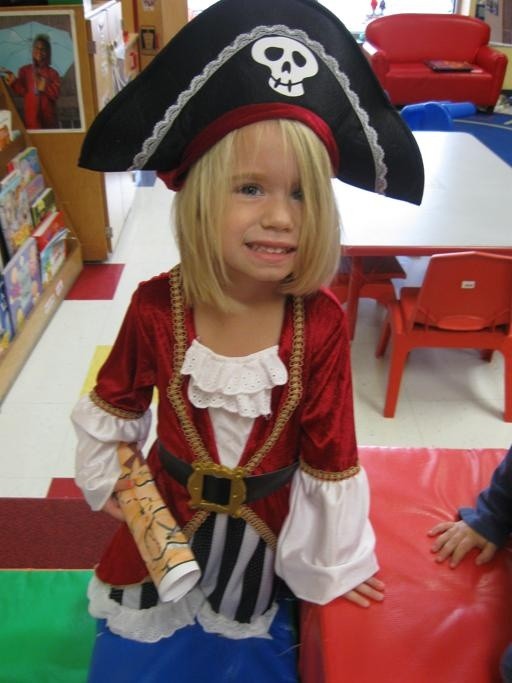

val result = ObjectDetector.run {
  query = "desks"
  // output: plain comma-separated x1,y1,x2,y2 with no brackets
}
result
330,131,512,256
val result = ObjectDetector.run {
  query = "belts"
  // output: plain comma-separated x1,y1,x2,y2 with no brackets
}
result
158,443,300,516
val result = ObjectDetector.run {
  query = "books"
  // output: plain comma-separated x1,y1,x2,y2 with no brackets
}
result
0,107,75,356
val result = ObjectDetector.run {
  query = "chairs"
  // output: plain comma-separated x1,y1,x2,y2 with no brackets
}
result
400,101,453,130
331,257,406,343
376,251,512,422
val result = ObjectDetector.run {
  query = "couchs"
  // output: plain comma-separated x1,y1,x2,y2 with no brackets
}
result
365,12,508,114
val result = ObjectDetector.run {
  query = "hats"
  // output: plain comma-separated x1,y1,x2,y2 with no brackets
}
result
78,0,425,206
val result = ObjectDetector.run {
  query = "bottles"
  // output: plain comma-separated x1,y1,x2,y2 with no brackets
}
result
377,0,386,16
359,0,379,41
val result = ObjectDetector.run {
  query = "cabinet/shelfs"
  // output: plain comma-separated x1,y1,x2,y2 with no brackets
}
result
0,85,85,410
0,0,141,262
92,0,188,81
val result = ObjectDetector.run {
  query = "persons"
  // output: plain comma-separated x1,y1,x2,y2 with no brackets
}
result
0,34,63,129
428,442,512,682
68,119,383,679
